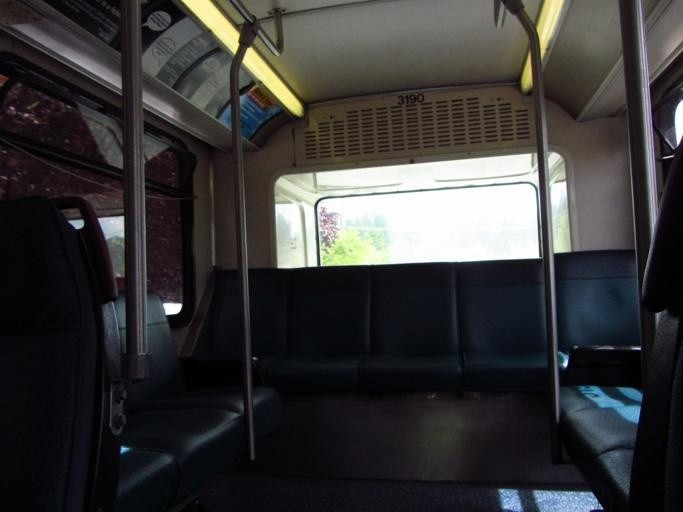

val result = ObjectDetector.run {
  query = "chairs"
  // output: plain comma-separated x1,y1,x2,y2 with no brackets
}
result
457,258,551,389
1,194,288,512
365,261,463,390
285,265,369,388
553,135,683,511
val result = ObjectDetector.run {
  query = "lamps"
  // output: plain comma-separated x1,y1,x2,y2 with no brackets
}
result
516,0,571,96
177,1,308,123
